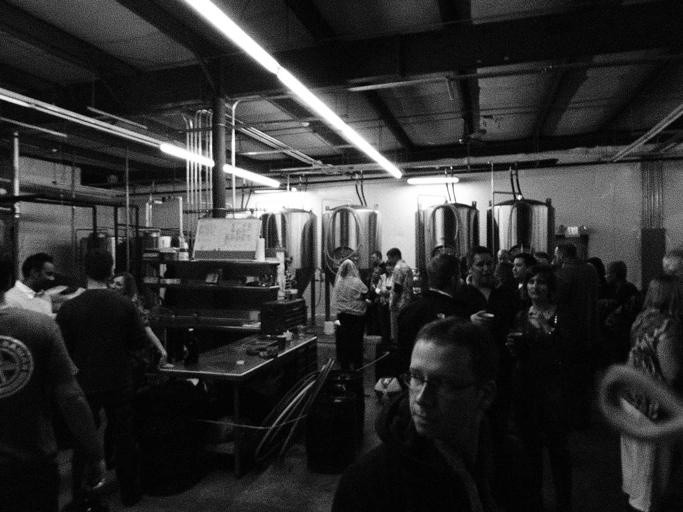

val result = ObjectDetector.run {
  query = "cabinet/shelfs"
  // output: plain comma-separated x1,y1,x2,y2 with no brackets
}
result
140,253,286,334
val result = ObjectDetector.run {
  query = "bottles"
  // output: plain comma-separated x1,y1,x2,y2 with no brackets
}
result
182,328,199,368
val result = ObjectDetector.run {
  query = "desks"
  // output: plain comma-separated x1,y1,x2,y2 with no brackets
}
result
158,331,318,479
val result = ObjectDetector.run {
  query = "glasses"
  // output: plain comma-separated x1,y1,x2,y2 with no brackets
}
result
399,371,475,394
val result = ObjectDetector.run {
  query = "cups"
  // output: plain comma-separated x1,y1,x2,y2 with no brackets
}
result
298,324,306,340
158,235,170,252
275,335,286,353
508,332,524,357
481,312,494,319
229,344,246,367
256,336,268,352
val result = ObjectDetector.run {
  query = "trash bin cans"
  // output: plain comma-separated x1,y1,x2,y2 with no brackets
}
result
363,335,382,360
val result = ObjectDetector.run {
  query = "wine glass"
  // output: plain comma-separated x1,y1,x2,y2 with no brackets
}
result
385,278,392,296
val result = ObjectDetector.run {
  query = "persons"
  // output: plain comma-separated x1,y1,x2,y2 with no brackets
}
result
330,243,683,512
0,248,169,512
